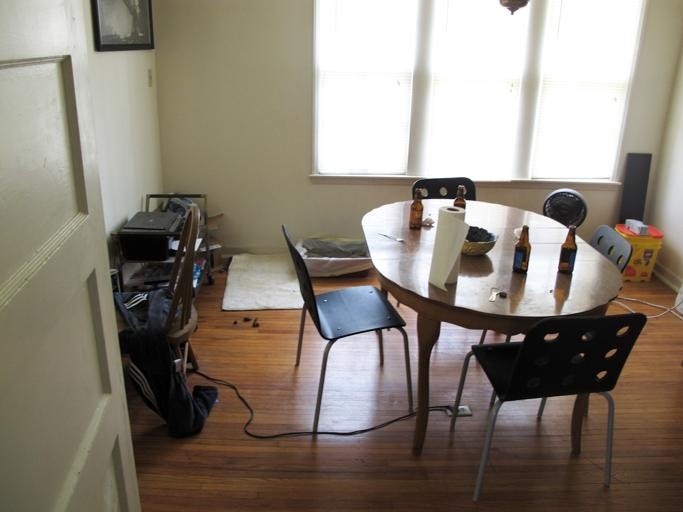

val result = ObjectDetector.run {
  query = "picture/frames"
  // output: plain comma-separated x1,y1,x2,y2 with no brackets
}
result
91,0,154,52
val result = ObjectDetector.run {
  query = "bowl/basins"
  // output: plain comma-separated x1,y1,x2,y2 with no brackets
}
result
460,225,497,256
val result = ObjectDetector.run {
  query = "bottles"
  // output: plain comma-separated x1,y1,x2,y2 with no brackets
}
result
453,185,466,210
409,190,423,231
511,225,530,272
557,224,577,274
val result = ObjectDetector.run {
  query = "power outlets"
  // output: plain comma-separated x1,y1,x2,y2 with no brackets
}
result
446,405,472,416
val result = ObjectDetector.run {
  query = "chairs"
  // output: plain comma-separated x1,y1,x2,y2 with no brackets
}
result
479,225,632,408
282,223,413,438
451,313,647,503
412,177,476,200
161,204,201,373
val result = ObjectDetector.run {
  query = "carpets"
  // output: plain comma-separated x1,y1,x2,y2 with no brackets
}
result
221,253,305,311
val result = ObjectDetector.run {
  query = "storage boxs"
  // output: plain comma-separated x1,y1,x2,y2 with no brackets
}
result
118,211,182,261
614,223,663,282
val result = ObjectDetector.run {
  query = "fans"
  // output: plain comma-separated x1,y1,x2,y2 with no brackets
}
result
542,188,587,230
